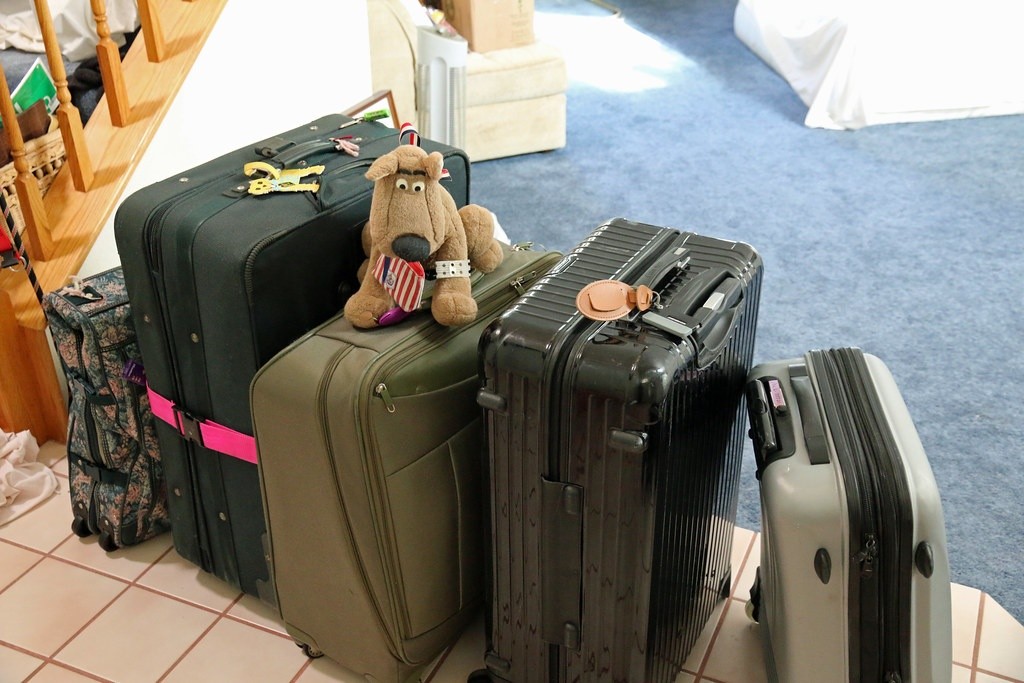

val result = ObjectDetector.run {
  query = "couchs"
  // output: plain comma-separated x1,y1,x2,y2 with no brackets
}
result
368,0,566,164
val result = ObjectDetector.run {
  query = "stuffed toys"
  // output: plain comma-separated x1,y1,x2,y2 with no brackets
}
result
343,144,504,329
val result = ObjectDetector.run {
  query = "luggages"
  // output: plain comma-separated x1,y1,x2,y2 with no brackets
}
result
746,348,954,683
111,112,473,598
466,216,767,683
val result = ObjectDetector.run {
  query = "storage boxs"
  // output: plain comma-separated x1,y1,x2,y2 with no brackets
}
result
441,1,534,54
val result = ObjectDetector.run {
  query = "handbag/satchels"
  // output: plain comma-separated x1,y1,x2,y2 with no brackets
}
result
40,267,173,552
247,236,557,683
